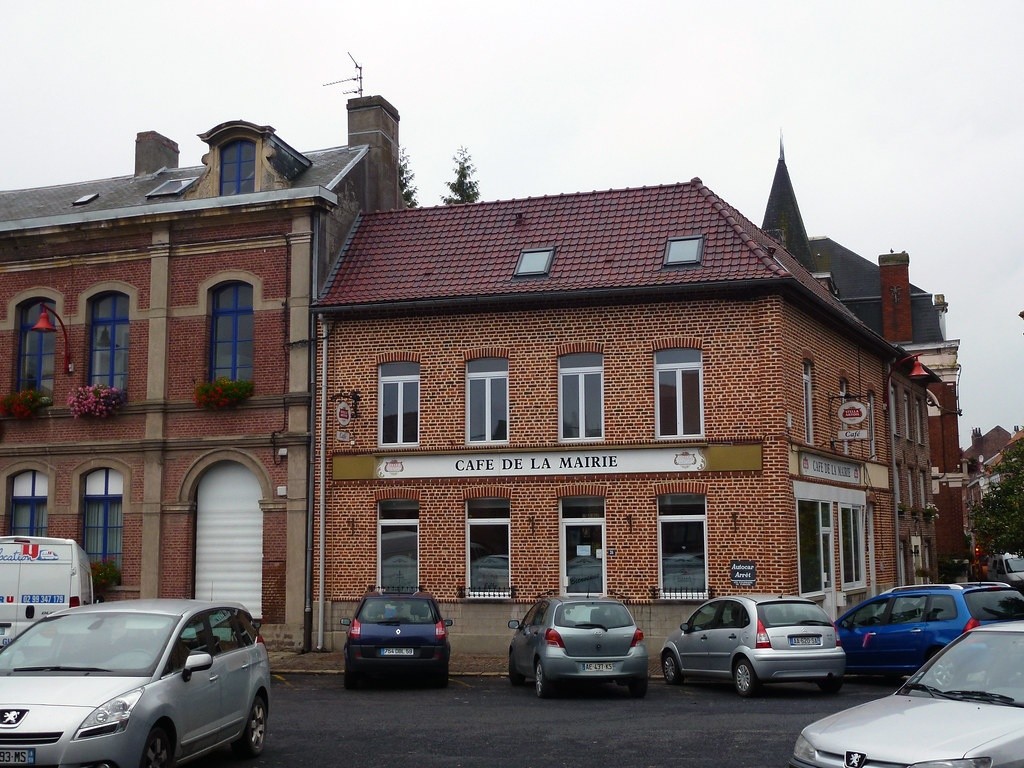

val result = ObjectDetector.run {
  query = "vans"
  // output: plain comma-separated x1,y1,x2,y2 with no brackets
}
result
0,535,104,651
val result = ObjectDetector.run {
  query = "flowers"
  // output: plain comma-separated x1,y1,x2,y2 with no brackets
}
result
67,384,127,422
191,376,255,413
0,390,52,418
90,556,120,590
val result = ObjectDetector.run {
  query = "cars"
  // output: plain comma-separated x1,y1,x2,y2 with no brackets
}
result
506,596,651,700
784,620,1024,768
339,584,453,690
0,597,273,768
658,593,848,698
986,552,1024,596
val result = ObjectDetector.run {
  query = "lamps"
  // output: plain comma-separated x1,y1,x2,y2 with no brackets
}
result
884,351,930,411
31,304,71,373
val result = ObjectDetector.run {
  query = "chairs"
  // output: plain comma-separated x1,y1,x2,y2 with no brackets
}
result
589,609,603,623
726,607,748,625
410,605,424,620
371,602,384,618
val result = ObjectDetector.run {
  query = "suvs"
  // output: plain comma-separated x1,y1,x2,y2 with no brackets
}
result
830,580,1024,685
